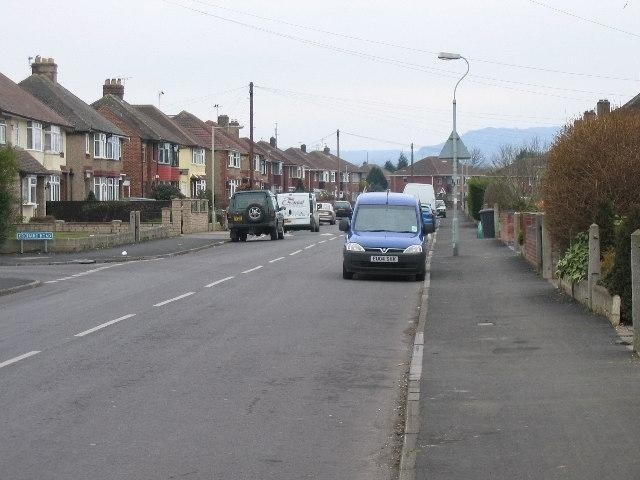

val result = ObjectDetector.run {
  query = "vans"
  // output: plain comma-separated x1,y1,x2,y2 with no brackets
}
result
402,183,436,210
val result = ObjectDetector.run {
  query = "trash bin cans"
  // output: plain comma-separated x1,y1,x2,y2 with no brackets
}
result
478,209,495,238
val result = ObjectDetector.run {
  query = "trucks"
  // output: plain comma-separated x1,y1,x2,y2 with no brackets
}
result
277,193,322,232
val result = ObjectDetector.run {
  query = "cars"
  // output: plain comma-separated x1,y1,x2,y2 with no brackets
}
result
420,204,436,233
435,200,447,218
339,192,426,280
333,201,354,220
317,203,336,225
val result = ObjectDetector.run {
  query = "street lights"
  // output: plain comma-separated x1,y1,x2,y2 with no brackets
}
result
438,52,469,255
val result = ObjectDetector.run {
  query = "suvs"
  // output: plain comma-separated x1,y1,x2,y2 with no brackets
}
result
227,190,285,242
